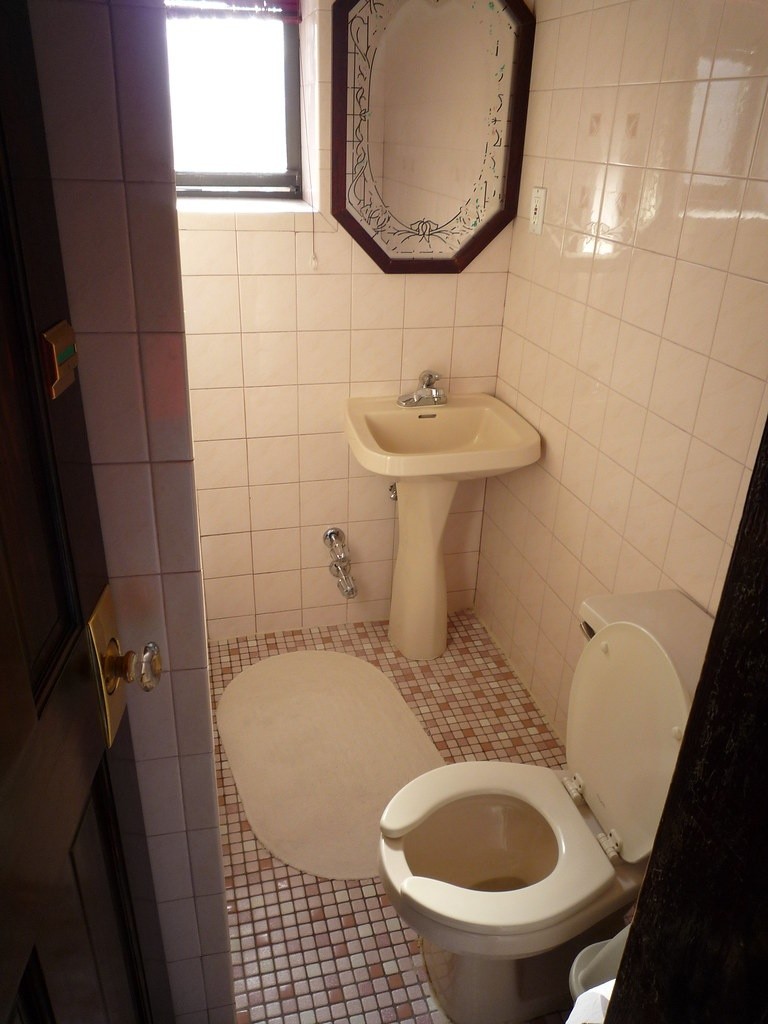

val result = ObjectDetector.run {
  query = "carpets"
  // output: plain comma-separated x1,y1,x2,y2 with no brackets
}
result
215,651,448,881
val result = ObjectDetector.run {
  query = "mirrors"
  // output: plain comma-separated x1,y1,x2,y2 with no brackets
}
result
332,0,537,274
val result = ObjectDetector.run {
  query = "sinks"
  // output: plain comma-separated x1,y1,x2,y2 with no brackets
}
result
343,392,543,480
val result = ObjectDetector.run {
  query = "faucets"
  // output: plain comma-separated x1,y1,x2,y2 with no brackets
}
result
396,368,448,408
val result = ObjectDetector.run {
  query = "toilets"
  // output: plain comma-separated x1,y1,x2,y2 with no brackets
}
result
375,583,720,1024
